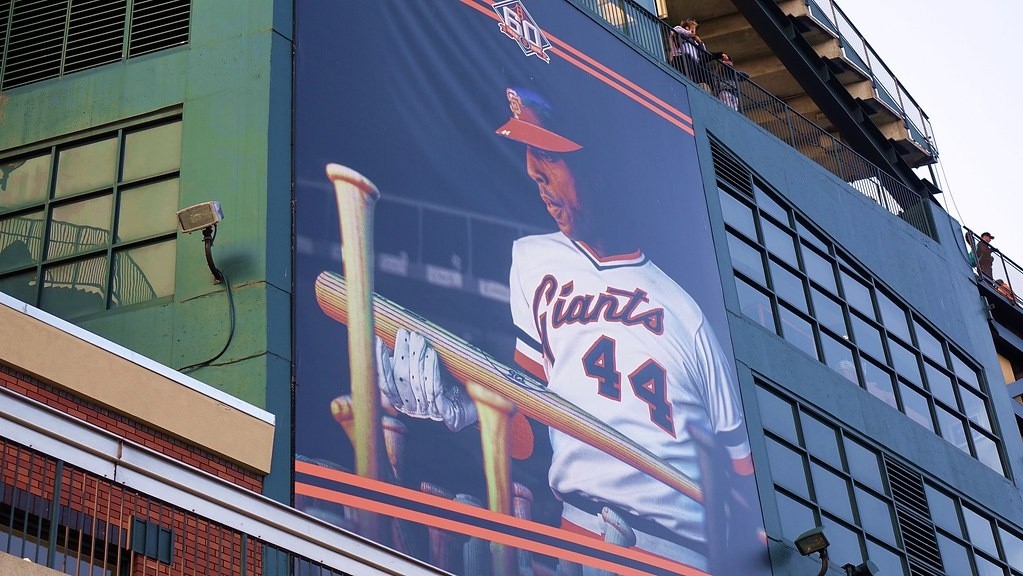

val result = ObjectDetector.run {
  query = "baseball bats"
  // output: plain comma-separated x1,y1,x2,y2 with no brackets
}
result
315,270,703,505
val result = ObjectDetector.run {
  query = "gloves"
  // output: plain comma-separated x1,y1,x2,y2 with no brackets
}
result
370,329,480,433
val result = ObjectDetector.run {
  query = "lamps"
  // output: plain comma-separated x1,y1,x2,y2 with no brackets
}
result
794,525,880,576
175,201,226,284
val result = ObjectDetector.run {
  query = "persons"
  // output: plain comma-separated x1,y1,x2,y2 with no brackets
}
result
375,85,760,576
995,279,1018,305
976,232,998,286
669,18,728,97
716,56,749,112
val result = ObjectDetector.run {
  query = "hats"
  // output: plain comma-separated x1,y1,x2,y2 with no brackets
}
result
495,86,586,153
981,232,994,240
